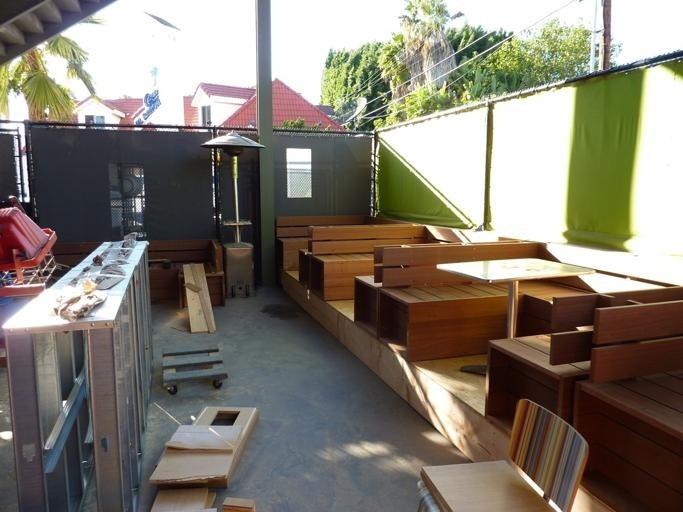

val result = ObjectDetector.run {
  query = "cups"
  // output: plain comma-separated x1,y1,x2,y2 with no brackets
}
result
84,114,95,130
95,116,105,130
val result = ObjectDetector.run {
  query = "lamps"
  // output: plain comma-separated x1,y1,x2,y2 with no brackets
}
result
201,130,266,297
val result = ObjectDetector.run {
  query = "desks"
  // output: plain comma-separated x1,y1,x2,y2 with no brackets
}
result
436,257,596,377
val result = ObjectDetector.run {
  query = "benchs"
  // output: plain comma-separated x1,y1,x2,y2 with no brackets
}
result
484,286,683,423
572,299,683,512
276,214,366,269
299,224,425,299
354,239,599,361
52,239,225,308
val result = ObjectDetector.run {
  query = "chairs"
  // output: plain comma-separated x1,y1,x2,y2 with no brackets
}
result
417,399,589,512
